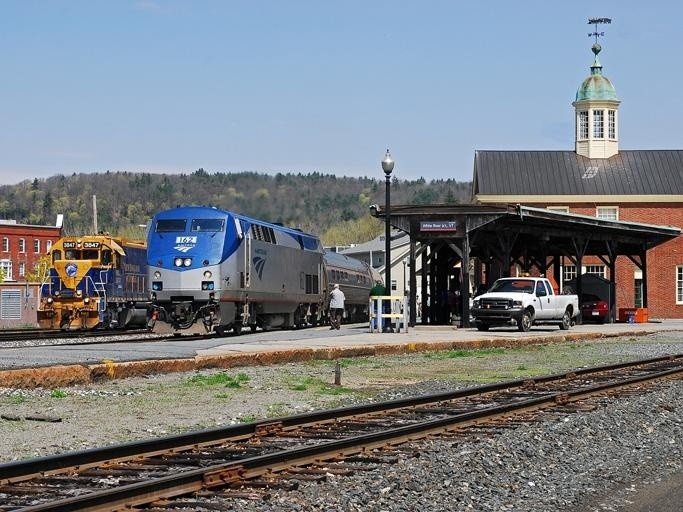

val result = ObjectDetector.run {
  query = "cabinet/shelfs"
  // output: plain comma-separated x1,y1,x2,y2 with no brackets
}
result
617,307,648,323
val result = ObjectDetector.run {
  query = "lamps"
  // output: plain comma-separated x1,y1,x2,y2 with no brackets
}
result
368,203,381,218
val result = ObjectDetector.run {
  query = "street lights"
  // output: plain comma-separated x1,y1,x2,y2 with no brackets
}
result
380,146,397,335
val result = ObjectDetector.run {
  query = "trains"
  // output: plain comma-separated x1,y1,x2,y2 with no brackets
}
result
140,207,383,333
34,231,155,334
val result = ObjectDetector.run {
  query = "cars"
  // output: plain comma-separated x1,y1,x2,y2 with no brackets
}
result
576,293,608,324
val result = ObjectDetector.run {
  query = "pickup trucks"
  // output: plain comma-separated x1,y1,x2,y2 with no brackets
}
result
466,272,585,332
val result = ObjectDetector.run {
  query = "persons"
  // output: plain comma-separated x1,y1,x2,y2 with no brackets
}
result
329,284,346,331
438,290,462,324
369,280,386,328
